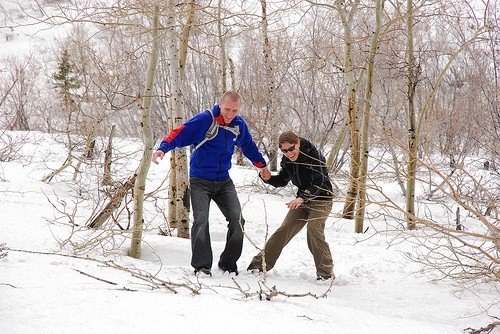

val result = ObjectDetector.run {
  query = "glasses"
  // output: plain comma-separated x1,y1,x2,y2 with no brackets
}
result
282,144,295,152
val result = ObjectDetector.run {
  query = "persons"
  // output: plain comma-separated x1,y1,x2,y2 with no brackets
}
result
153,90,272,279
249,130,335,282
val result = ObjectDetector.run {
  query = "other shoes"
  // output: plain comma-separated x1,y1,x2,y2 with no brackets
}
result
195,267,211,278
217,261,239,277
315,276,335,282
